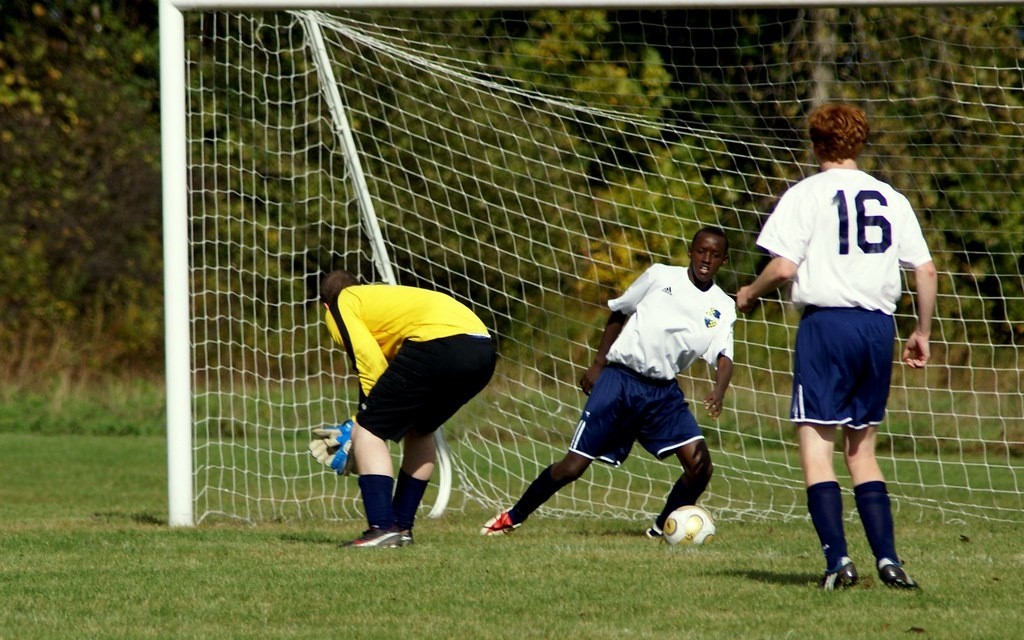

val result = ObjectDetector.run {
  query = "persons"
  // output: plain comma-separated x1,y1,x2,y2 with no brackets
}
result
321,269,497,549
481,225,737,538
737,104,938,592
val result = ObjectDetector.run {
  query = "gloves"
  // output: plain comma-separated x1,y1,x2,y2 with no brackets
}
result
309,415,356,467
323,435,360,476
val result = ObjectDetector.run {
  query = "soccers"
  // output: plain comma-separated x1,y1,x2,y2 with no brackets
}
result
663,504,717,545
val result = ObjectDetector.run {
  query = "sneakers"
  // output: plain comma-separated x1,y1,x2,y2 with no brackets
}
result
820,556,858,592
647,523,663,538
338,525,402,549
392,525,412,544
480,507,522,536
880,564,923,590
